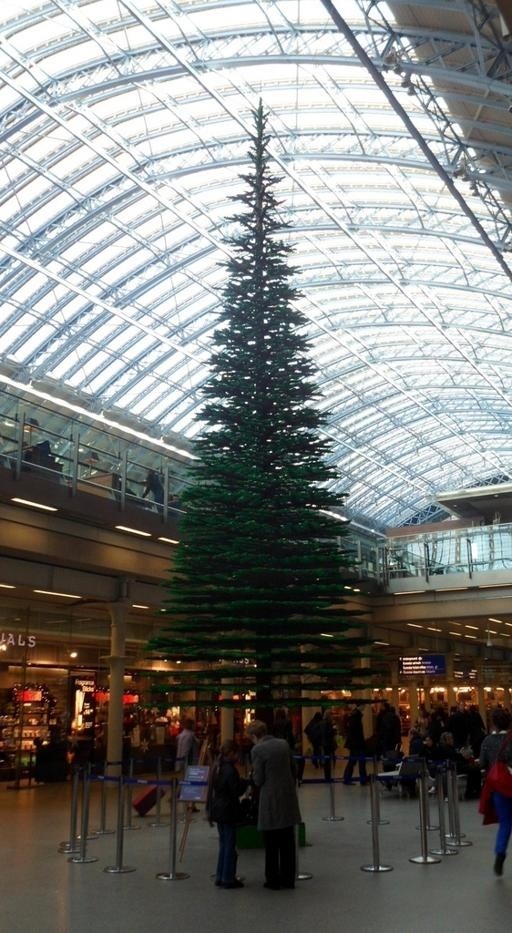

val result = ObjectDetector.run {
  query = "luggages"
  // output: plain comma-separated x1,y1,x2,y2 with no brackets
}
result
132,775,182,817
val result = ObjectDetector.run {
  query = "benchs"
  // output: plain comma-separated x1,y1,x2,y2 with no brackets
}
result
2,439,64,478
378,748,485,797
69,471,151,510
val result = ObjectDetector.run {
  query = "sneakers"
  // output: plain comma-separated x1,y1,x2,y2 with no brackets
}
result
214,879,244,889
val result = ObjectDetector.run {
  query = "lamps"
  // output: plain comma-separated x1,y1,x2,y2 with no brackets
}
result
21,416,42,434
83,450,99,477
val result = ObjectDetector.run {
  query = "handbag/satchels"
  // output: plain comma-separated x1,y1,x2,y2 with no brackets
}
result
487,760,512,798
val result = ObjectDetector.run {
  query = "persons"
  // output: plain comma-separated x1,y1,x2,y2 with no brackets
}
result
207,738,253,887
176,700,512,797
142,468,169,514
479,707,511,876
244,719,302,891
